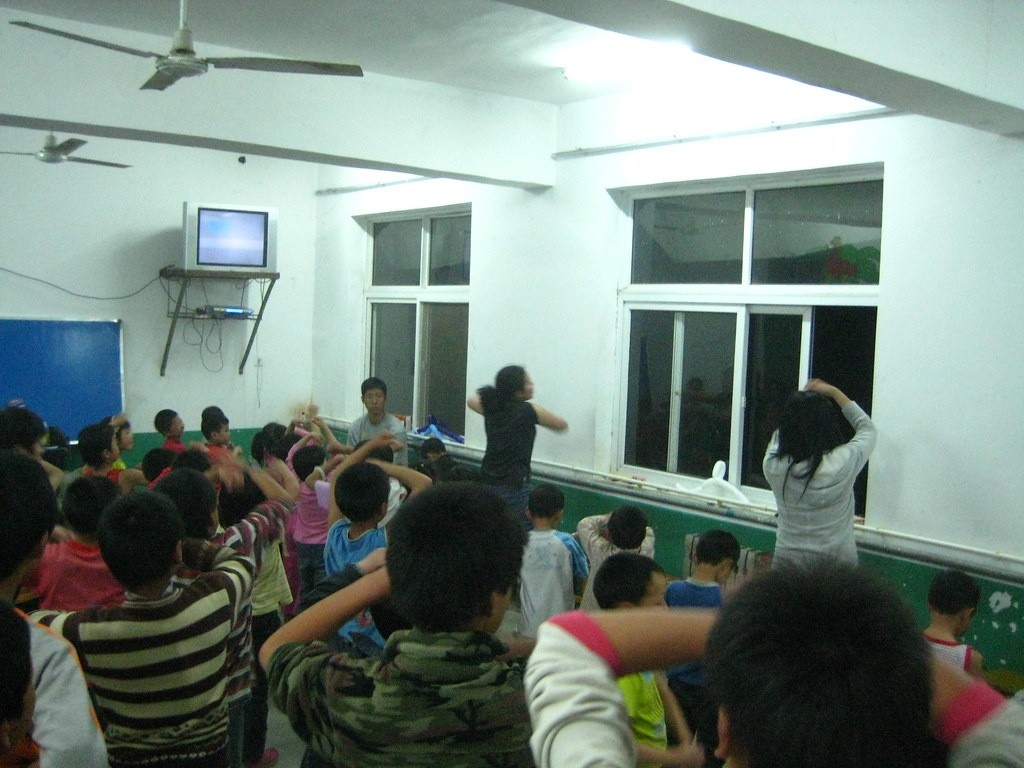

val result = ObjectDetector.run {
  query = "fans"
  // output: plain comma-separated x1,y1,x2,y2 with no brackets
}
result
8,0,364,89
0,128,132,168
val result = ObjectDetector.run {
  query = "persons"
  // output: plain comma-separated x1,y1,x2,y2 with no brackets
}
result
762,377,877,573
467,365,570,533
344,377,409,467
0,401,1024,768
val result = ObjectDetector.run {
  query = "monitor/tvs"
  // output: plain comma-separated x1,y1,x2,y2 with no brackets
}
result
180,201,277,274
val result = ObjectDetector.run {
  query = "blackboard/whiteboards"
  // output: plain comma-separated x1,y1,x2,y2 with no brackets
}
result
0,316,126,450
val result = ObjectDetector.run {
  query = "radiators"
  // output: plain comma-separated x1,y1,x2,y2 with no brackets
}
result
681,531,762,590
